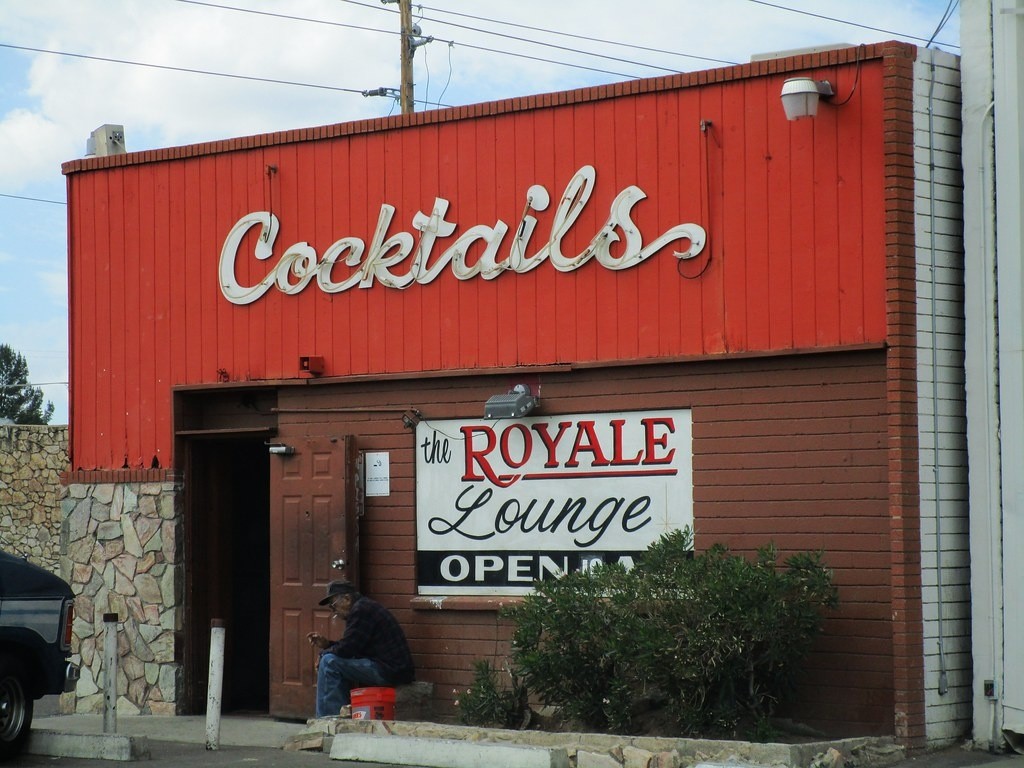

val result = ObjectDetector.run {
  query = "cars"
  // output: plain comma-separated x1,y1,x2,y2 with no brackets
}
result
0,551,80,765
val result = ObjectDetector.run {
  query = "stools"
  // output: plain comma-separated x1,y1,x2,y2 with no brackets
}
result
392,681,433,720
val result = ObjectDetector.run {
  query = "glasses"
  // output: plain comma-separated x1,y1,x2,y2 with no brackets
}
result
328,595,344,608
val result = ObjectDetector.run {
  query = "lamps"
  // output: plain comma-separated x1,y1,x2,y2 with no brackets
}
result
779,77,835,120
484,384,539,420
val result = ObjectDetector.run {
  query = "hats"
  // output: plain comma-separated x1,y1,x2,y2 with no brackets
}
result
319,580,356,606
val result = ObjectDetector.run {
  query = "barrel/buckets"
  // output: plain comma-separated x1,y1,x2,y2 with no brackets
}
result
350,687,396,721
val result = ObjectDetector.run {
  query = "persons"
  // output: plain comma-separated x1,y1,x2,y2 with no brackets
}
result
306,580,416,719
